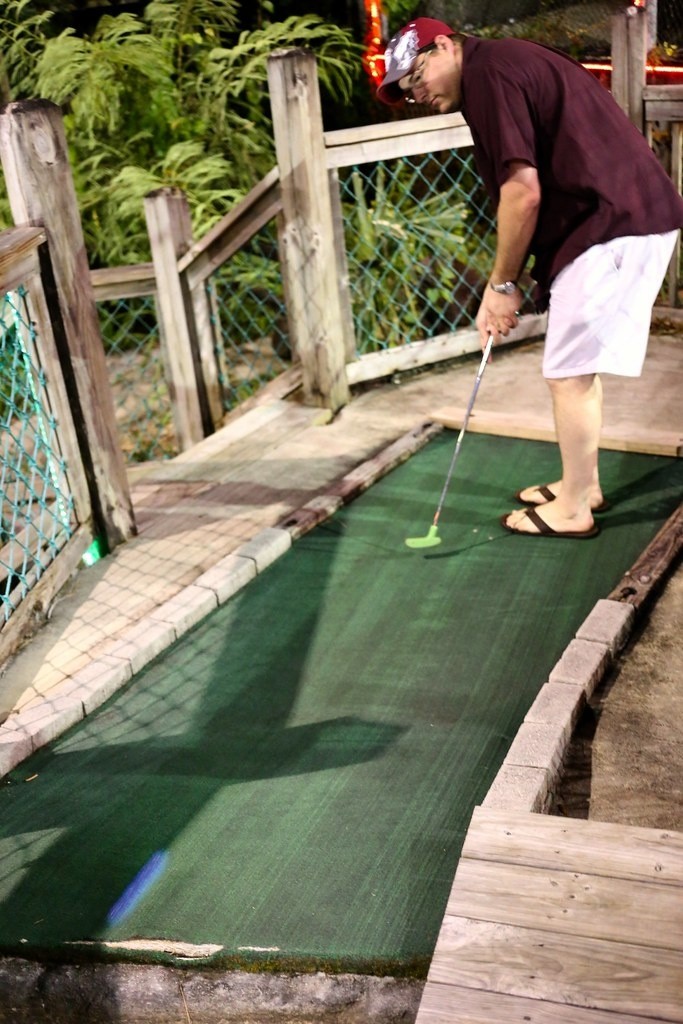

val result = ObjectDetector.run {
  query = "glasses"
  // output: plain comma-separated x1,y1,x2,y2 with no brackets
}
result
404,48,434,104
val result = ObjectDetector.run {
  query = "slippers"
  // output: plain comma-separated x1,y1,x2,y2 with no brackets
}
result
515,483,611,513
501,506,599,540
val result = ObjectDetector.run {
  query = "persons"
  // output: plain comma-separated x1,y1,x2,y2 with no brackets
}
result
377,18,683,539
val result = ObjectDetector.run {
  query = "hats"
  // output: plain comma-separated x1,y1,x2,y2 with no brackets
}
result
376,17,459,108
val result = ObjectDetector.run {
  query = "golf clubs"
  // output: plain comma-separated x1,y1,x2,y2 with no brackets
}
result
404,331,495,550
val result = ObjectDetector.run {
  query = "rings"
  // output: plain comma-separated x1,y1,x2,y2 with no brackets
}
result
515,311,519,315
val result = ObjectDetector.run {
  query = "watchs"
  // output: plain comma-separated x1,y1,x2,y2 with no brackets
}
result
489,277,518,295
